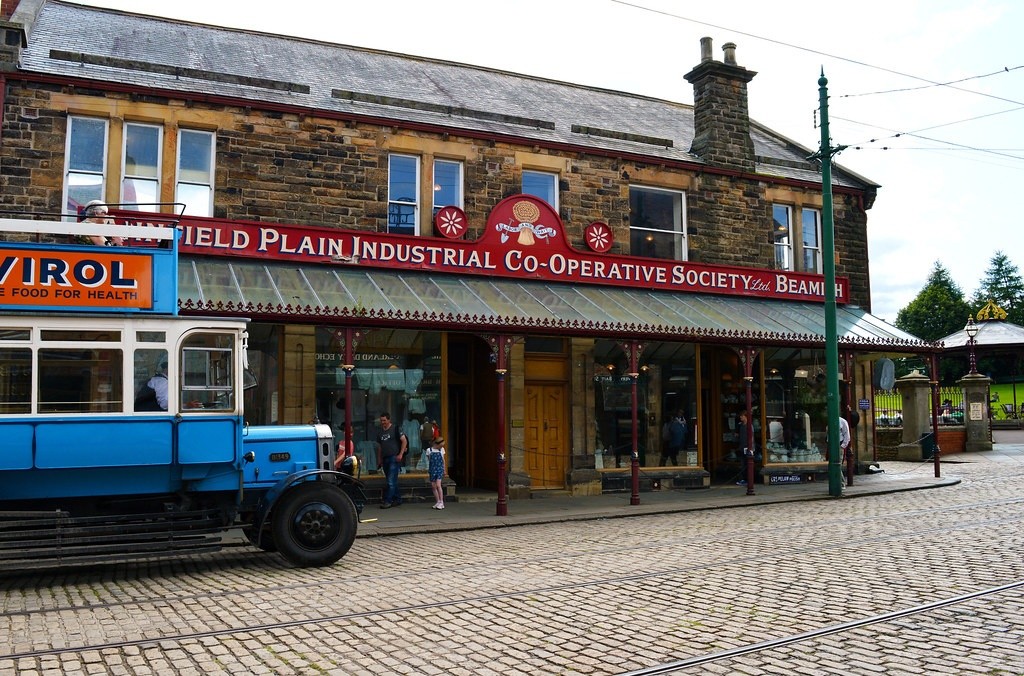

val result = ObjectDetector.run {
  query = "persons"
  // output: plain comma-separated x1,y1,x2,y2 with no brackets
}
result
377,412,407,507
658,408,687,467
74,200,110,245
426,438,446,509
735,411,755,486
770,419,784,444
882,410,890,425
891,411,902,426
420,417,440,451
990,392,1000,402
334,425,354,471
106,224,125,246
942,399,964,414
825,417,850,488
133,361,203,412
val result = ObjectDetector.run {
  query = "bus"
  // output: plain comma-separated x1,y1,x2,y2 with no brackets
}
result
0,199,380,566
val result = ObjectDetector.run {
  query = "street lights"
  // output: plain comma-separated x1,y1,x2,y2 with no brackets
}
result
963,314,987,378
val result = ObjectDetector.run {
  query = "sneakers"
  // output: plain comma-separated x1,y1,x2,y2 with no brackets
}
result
437,503,444,509
431,502,440,509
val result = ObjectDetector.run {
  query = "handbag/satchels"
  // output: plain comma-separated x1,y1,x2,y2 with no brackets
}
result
395,425,409,457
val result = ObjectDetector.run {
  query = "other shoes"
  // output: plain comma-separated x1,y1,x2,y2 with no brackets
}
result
841,482,846,489
391,501,402,507
379,502,392,509
736,480,748,486
